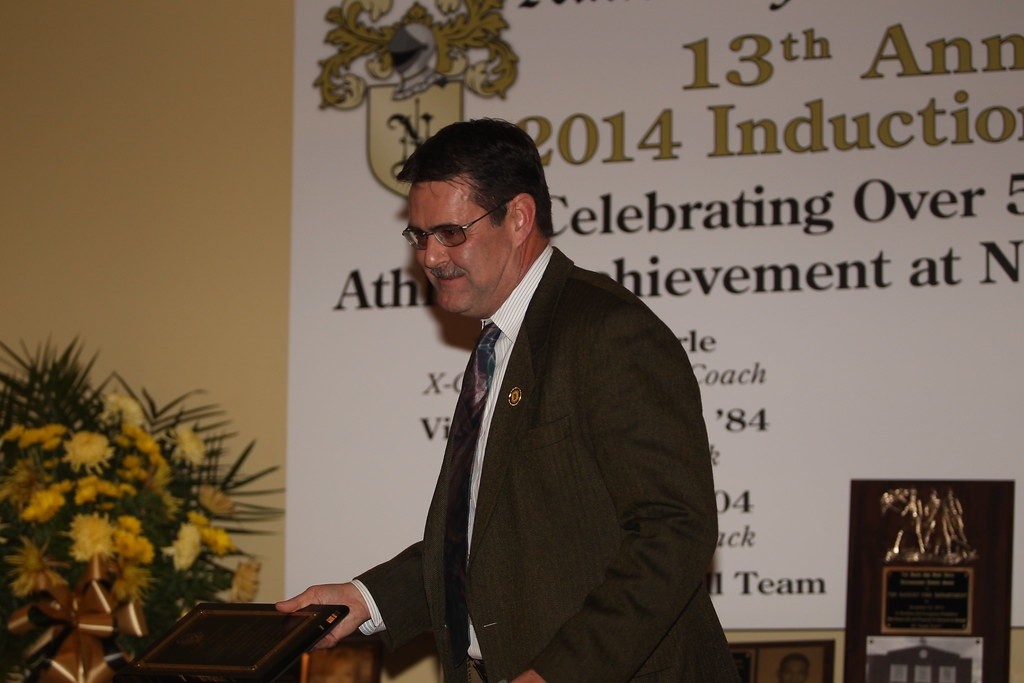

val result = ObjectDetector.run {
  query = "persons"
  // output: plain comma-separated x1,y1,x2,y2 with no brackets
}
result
884,486,965,560
777,653,809,683
274,118,740,683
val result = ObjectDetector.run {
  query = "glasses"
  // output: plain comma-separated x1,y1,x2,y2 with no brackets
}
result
401,197,513,251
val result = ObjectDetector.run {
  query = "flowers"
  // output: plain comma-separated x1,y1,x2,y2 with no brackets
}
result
0,334,288,651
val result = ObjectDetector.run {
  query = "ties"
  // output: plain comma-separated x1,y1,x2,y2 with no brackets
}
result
442,320,501,667
